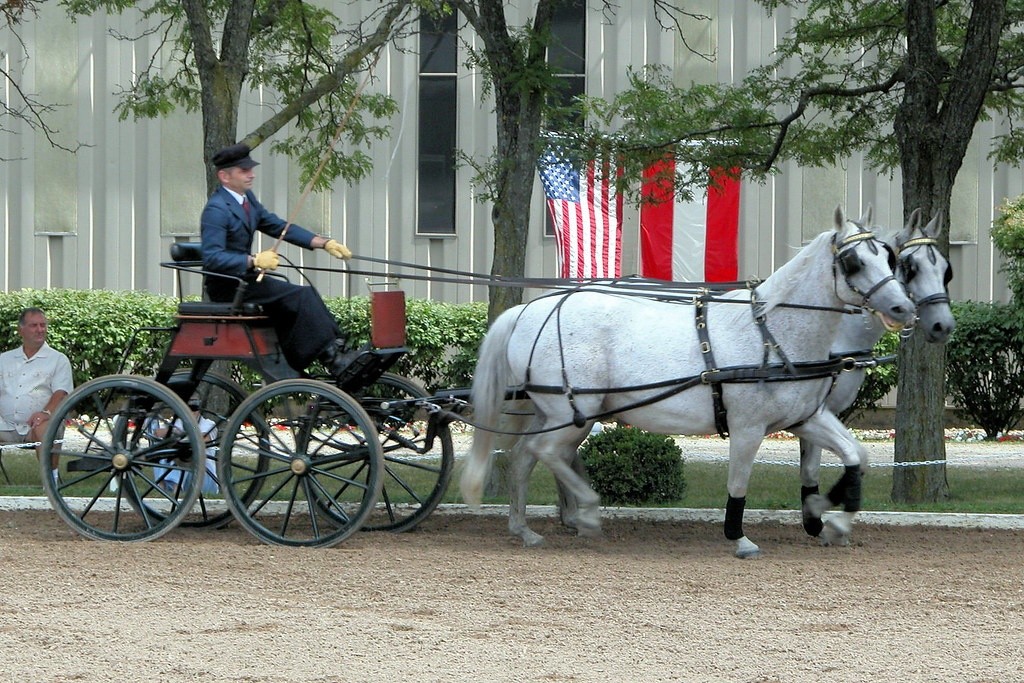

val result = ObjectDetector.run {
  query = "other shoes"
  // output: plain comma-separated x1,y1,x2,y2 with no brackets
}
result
337,343,367,379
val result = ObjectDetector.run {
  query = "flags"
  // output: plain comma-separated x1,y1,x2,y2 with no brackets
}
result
639,140,739,284
538,132,623,281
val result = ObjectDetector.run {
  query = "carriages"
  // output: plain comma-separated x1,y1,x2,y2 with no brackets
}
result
40,201,960,558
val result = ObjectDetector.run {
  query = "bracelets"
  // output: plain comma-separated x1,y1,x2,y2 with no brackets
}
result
42,411,50,415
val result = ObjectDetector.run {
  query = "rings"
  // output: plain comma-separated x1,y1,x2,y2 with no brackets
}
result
37,418,39,420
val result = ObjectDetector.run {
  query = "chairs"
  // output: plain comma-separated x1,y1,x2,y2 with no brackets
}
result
160,243,290,317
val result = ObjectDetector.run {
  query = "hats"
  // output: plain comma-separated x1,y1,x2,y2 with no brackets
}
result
208,143,261,172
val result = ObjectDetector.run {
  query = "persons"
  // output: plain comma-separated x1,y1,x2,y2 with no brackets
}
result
201,143,369,377
0,308,73,494
153,389,219,497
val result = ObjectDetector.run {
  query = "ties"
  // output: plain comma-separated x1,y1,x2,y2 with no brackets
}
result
241,196,251,212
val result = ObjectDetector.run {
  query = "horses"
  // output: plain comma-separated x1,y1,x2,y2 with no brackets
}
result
456,204,955,563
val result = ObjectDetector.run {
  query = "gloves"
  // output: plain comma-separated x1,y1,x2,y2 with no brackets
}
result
253,250,282,271
325,241,352,262
